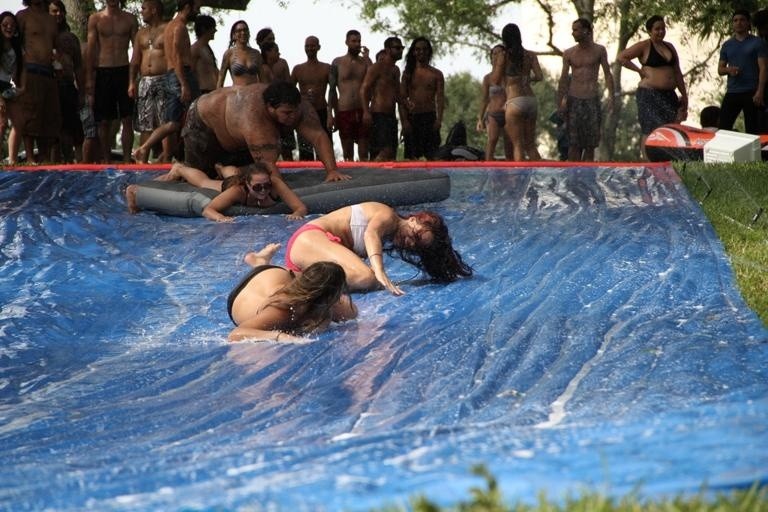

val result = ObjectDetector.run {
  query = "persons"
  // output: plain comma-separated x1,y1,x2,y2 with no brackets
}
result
226,240,357,347
557,19,614,163
158,160,308,222
617,16,687,159
717,9,765,135
477,24,542,162
126,83,352,216
284,200,461,296
1,1,444,165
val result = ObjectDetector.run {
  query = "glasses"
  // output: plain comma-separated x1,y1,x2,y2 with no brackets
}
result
1,22,18,27
232,28,248,33
389,46,405,50
249,182,271,191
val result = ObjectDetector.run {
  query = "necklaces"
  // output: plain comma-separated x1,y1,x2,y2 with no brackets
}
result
257,200,261,206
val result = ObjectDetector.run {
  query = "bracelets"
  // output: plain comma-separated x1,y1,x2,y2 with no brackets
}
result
368,253,383,260
273,331,284,342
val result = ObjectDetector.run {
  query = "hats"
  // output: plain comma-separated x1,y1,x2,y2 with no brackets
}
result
733,9,753,31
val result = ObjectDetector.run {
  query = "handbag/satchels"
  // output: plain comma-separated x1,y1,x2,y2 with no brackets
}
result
452,120,467,145
